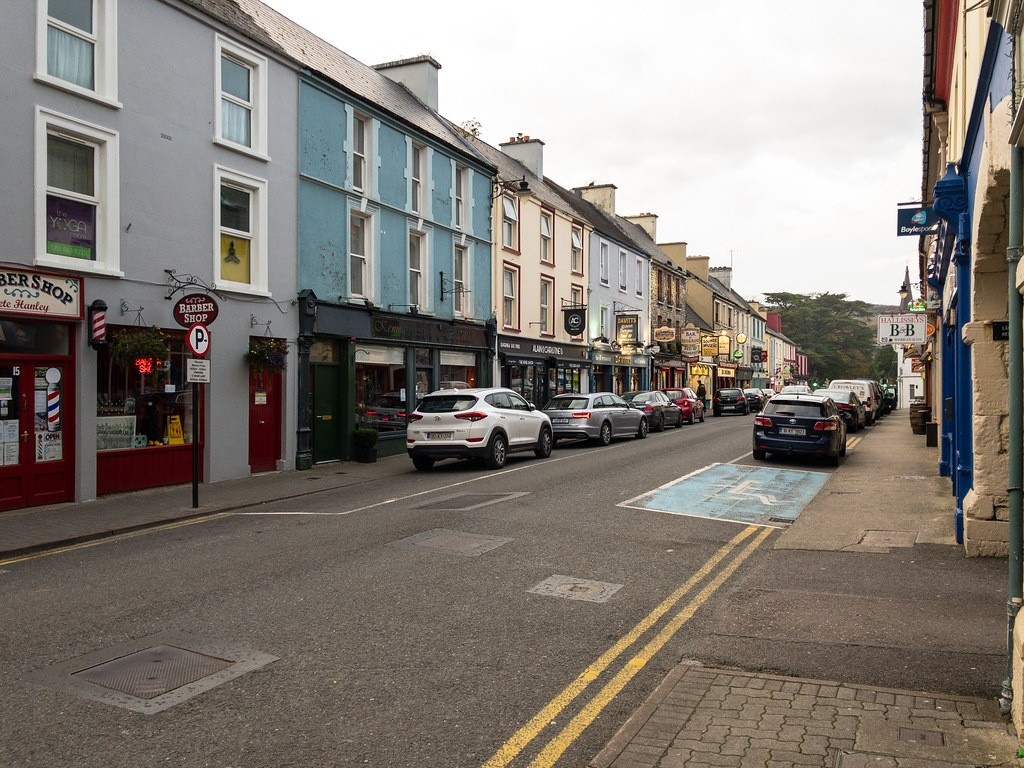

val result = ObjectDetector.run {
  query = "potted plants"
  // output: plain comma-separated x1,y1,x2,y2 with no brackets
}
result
351,428,378,464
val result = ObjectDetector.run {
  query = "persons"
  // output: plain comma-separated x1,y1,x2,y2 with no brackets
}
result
696,380,706,412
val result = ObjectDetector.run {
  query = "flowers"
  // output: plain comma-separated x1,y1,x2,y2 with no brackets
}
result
113,325,171,392
242,337,290,378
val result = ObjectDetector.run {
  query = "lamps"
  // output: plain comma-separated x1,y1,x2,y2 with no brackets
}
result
338,296,374,310
490,175,532,208
87,299,109,352
388,305,418,317
897,282,923,299
464,317,493,329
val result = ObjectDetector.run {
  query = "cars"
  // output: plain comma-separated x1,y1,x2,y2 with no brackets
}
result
752,390,847,467
135,390,193,443
713,387,776,417
620,387,705,432
780,379,897,434
362,380,472,430
540,392,649,446
406,388,554,472
